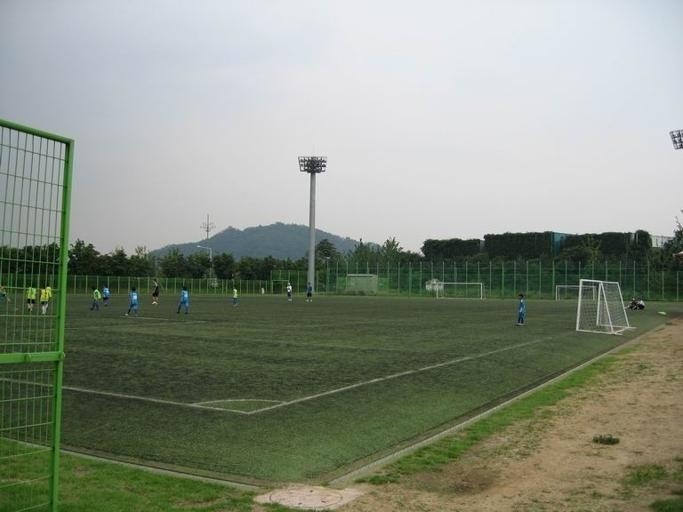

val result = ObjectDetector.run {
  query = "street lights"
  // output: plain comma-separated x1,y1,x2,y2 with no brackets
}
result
198,245,213,280
299,156,326,294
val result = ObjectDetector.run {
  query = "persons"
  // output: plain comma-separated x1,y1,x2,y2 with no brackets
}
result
102,285,110,306
286,283,293,302
152,279,158,305
24,285,52,316
624,297,645,310
124,287,139,316
175,287,189,315
304,281,313,303
232,284,239,307
513,294,526,326
88,286,102,311
0,285,13,304
